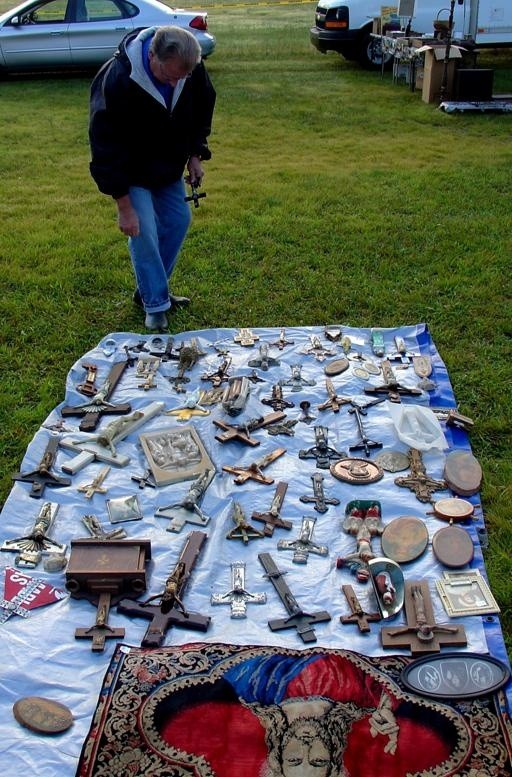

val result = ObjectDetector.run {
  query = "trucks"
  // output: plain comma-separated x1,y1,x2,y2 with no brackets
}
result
310,1,512,69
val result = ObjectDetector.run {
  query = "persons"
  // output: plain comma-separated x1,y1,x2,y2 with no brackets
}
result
85,23,218,332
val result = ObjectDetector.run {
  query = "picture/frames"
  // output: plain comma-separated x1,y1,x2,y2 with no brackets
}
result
435,575,501,618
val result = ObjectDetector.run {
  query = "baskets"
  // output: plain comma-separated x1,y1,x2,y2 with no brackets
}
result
433,9,455,31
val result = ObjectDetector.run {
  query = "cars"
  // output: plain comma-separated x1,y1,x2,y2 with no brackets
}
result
1,1,216,67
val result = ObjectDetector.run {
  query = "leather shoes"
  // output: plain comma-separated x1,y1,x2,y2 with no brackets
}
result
132,285,191,313
143,311,169,334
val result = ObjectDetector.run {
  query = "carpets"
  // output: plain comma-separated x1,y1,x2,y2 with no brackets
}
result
74,645,512,777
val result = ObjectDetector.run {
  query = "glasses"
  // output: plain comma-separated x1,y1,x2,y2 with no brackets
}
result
159,62,194,83
